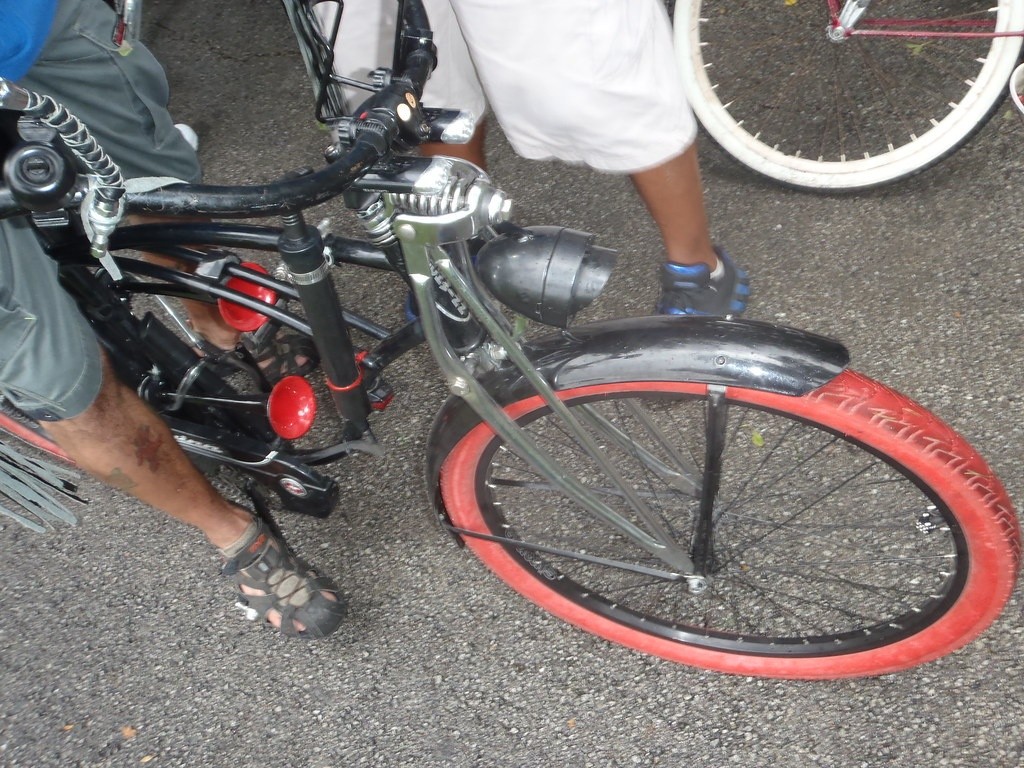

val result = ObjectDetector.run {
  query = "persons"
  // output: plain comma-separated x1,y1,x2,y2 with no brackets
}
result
0,0,349,637
287,0,750,317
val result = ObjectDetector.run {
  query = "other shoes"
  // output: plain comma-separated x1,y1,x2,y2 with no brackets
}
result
174,122,198,152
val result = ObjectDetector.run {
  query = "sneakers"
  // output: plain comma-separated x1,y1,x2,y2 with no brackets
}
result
404,248,477,325
653,245,752,318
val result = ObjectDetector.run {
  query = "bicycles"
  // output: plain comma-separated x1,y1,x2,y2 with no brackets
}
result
0,0,1024,684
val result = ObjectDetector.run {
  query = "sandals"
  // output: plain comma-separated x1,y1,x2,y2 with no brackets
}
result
189,323,319,385
216,514,347,640
